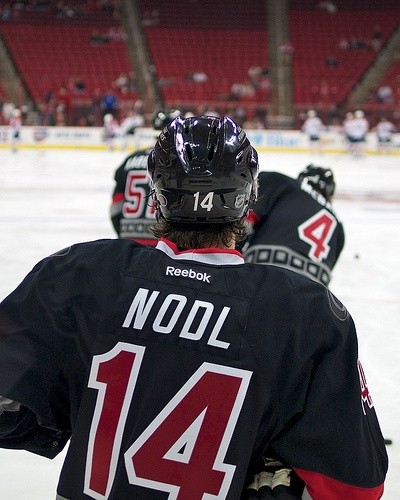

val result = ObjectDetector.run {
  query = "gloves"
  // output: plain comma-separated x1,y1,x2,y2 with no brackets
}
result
244,459,296,500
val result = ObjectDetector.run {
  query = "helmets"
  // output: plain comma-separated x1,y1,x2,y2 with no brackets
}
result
298,164,335,198
148,118,258,222
